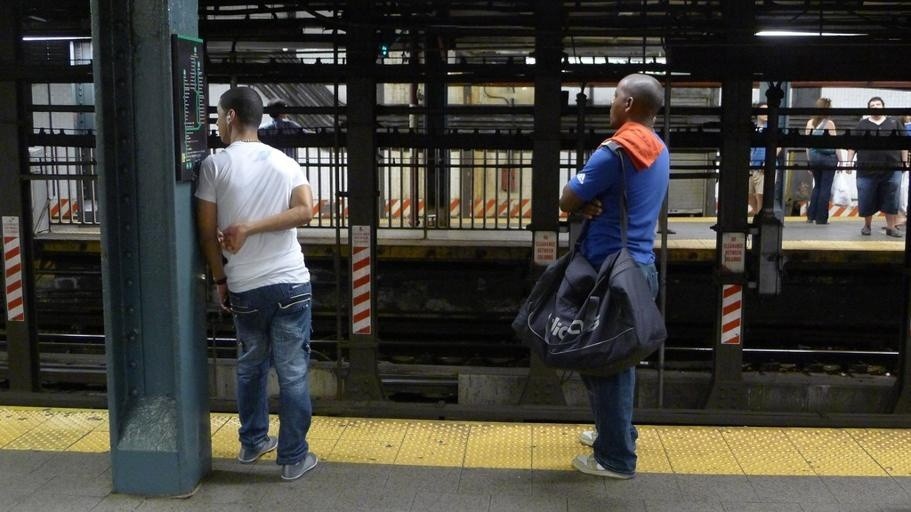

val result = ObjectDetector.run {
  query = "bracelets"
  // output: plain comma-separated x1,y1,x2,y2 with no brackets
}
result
216,276,227,285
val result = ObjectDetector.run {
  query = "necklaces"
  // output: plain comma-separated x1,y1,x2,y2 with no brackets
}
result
241,139,260,142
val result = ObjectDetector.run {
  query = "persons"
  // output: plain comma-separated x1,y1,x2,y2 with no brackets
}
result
845,96,908,238
196,87,318,480
749,102,782,216
560,74,669,479
805,97,844,225
895,115,911,220
257,99,316,163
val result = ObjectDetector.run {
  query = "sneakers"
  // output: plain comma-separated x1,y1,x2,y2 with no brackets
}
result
574,454,635,480
238,436,278,464
886,228,901,237
280,452,318,481
580,432,600,447
861,225,871,234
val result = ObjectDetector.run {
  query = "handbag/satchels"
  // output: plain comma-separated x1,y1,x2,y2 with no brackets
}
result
511,249,667,379
814,129,836,155
